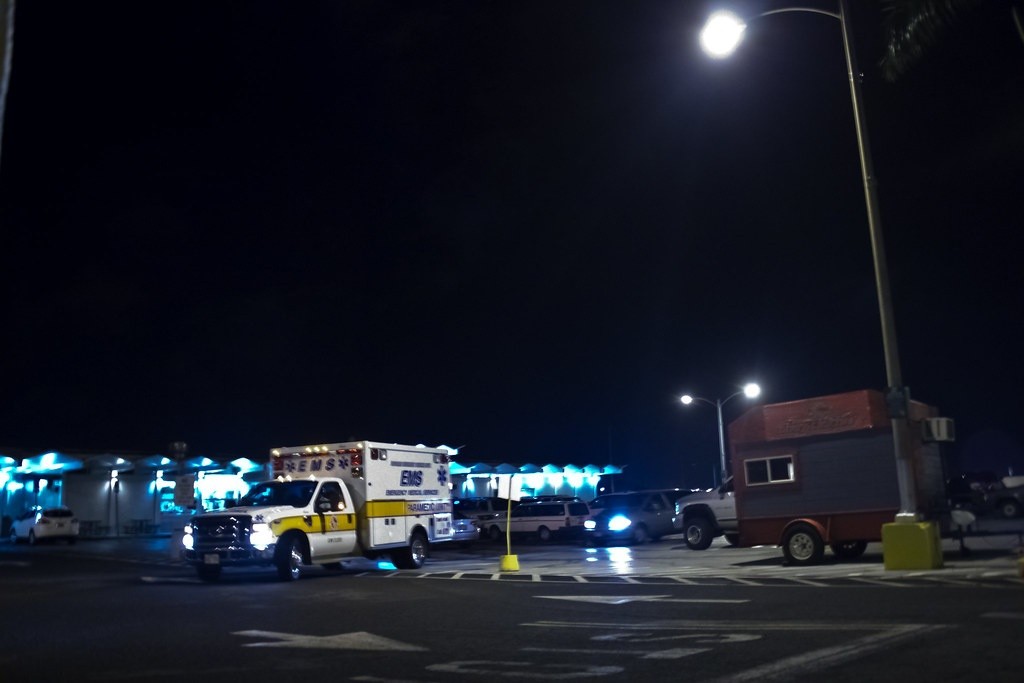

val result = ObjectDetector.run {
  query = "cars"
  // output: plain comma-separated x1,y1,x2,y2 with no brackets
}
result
454,496,521,539
675,475,742,550
982,484,1024,519
659,489,700,535
587,492,637,517
9,504,79,544
582,490,666,546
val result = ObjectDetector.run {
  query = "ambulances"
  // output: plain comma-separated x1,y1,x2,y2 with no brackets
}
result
178,440,459,584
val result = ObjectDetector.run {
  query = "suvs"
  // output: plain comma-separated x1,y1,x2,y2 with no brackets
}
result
482,495,589,542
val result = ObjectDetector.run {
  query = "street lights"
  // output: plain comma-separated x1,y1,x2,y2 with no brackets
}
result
678,380,762,484
500,473,519,571
701,8,937,574
